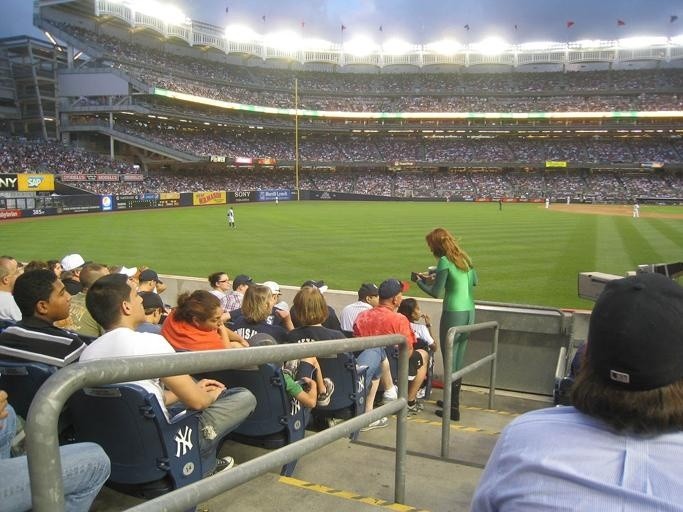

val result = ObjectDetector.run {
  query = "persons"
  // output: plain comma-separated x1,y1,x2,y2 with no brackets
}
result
2,254,437,511
226,206,237,230
468,269,681,511
630,202,641,218
1,14,681,208
411,229,477,421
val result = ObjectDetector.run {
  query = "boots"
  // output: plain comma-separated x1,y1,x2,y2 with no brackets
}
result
436,378,461,420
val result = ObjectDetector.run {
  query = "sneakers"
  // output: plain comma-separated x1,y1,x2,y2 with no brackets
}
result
212,456,234,475
360,384,432,431
317,377,334,406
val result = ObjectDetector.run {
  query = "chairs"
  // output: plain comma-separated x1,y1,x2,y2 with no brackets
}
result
386,347,399,385
60,383,203,490
314,353,369,442
1,361,58,420
202,363,306,481
413,338,434,400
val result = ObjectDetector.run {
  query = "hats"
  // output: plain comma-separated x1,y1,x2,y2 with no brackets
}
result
586,273,683,391
118,265,168,314
300,280,328,294
232,275,282,295
60,254,92,271
358,279,410,298
